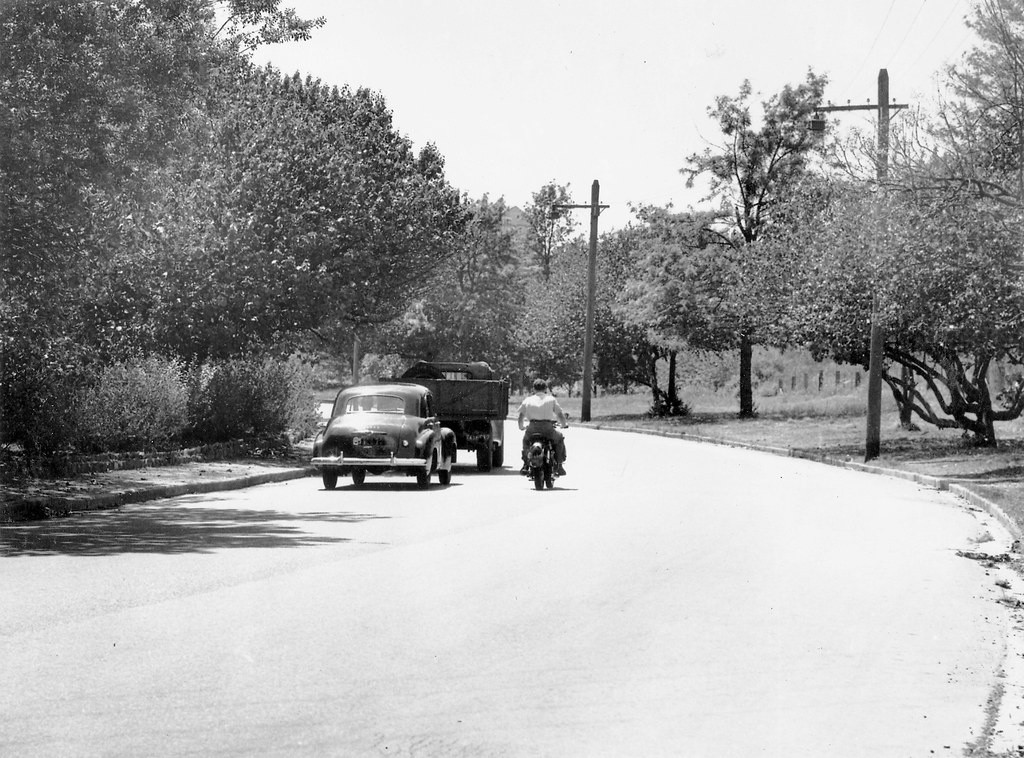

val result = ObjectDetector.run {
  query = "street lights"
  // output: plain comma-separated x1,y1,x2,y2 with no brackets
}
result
809,99,891,465
548,202,599,423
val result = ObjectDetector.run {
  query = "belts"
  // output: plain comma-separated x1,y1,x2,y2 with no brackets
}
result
530,420,550,422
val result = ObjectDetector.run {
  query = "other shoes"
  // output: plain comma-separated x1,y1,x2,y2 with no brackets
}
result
520,465,528,476
553,464,566,475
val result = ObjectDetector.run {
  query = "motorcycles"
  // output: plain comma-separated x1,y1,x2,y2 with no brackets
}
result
522,412,568,491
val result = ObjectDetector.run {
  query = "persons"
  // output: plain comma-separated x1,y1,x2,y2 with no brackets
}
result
516,378,569,475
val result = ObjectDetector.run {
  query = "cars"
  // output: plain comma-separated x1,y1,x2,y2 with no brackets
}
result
311,385,457,491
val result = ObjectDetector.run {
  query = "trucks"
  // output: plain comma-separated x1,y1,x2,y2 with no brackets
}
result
379,359,511,474
